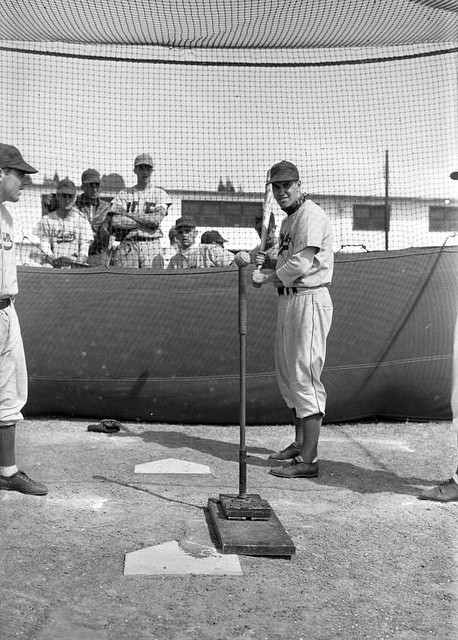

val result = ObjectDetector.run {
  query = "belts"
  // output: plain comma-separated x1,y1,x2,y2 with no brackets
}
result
277,286,309,296
0,297,10,310
89,250,104,254
123,236,159,242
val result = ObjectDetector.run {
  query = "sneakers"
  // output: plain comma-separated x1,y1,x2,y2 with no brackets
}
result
417,478,458,502
269,441,303,460
0,470,49,495
269,455,319,479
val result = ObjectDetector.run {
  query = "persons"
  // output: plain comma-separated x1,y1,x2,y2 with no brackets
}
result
0,142,49,498
250,211,279,263
163,225,180,269
199,230,229,250
250,161,336,478
108,153,173,272
33,179,94,269
167,215,235,270
44,168,111,267
418,171,458,502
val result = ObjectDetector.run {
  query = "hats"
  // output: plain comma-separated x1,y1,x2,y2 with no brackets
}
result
174,216,196,230
201,230,229,244
57,179,76,195
254,209,275,229
265,161,299,186
82,169,101,184
169,227,178,239
0,143,39,174
134,153,153,168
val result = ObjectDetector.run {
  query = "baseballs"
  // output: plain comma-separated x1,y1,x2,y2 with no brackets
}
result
235,251,251,267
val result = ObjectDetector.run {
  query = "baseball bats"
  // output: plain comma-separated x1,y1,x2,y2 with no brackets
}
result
253,169,274,287
